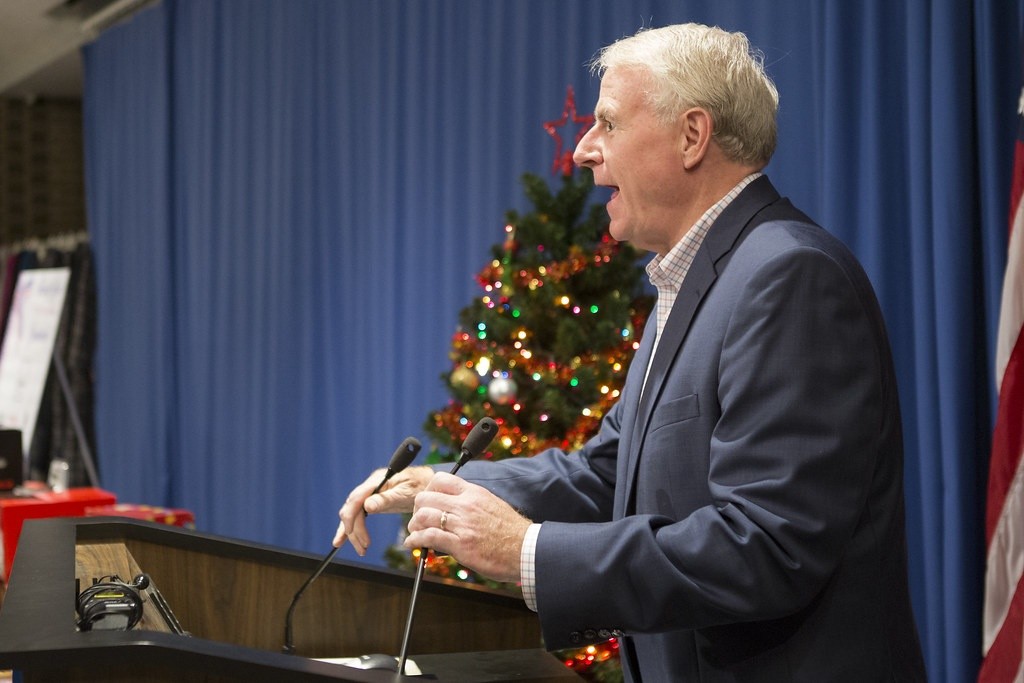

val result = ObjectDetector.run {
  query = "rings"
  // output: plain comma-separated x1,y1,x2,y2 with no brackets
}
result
439,511,450,531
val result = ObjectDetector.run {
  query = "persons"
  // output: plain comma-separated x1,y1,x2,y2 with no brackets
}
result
334,24,928,682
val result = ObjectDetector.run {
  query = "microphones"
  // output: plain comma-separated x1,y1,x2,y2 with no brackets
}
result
133,574,150,590
397,415,499,675
282,437,422,656
